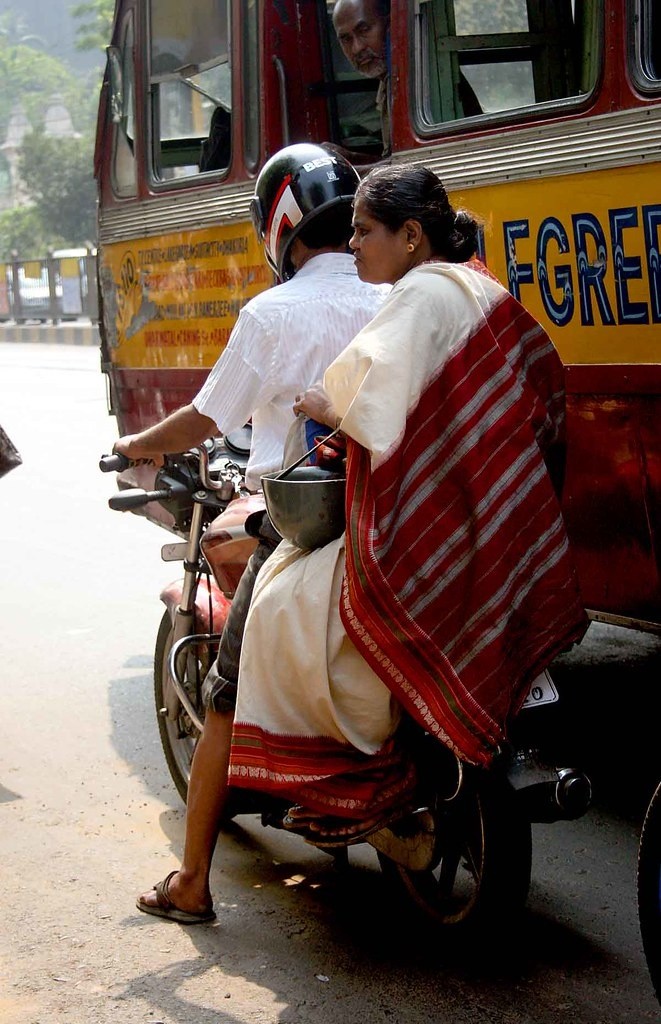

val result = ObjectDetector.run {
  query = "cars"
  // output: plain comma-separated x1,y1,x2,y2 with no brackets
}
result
6,267,77,323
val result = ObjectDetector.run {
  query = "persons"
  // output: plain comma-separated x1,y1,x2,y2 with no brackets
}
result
112,139,396,930
225,165,591,847
321,0,484,160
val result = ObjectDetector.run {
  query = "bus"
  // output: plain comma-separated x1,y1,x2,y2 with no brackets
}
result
93,2,661,632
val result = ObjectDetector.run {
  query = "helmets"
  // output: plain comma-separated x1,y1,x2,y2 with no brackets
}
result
260,427,346,551
246,143,361,282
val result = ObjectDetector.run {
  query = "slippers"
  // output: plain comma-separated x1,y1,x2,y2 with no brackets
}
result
137,869,219,923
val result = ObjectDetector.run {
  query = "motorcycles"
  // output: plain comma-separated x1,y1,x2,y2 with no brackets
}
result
100,418,596,958
